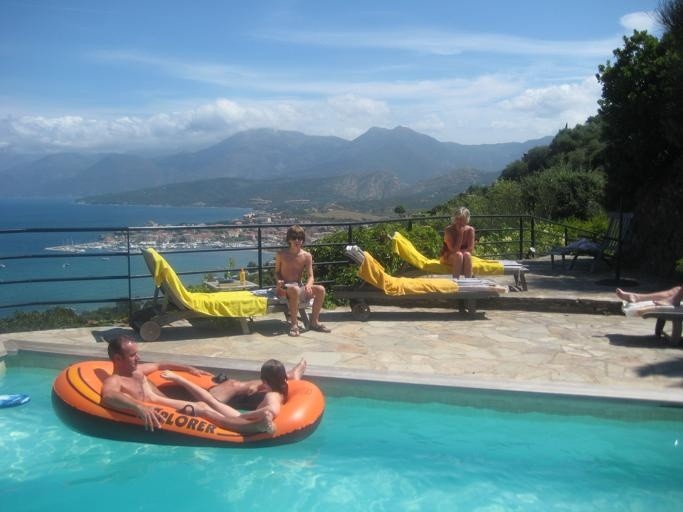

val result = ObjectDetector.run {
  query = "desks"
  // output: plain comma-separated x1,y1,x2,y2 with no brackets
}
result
203,278,261,326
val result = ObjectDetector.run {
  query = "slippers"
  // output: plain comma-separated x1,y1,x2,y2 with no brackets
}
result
289,326,300,337
310,324,330,332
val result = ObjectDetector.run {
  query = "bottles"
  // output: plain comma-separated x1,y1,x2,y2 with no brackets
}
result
240,268,245,285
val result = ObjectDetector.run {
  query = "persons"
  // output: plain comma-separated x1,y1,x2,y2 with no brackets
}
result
616,284,683,305
100,333,307,433
439,206,475,279
274,225,331,336
141,358,288,421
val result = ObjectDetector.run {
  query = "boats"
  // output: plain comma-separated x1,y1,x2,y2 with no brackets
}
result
50,362,325,448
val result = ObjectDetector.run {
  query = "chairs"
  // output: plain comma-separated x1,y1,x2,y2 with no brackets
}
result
548,211,636,274
620,299,682,343
389,232,532,293
333,241,509,321
134,247,311,338
